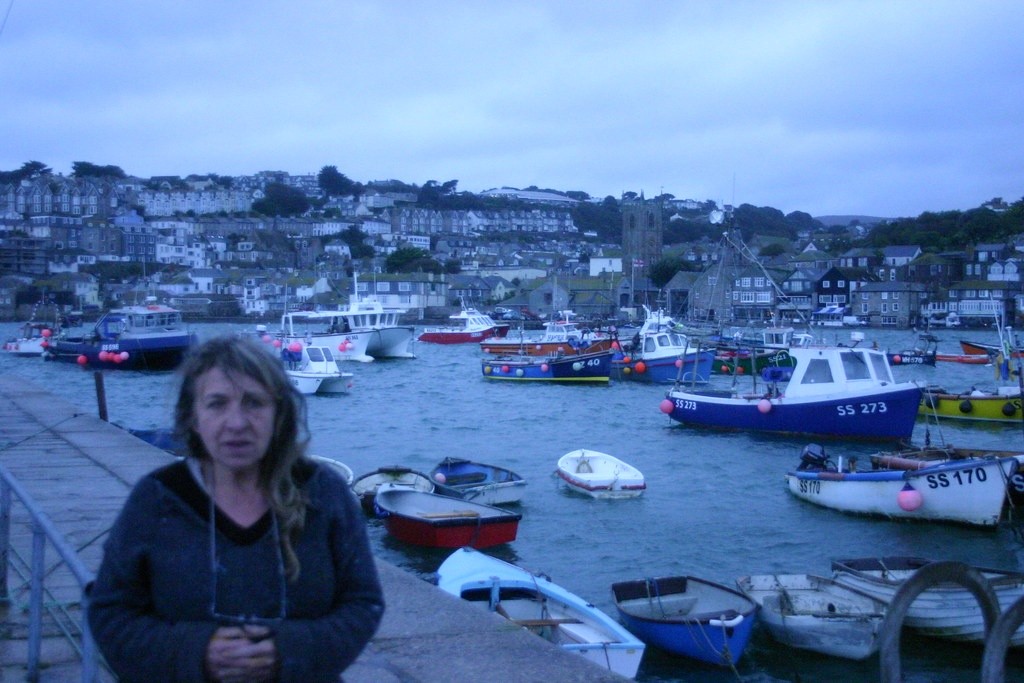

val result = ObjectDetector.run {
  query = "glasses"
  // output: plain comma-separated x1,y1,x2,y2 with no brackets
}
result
208,552,286,626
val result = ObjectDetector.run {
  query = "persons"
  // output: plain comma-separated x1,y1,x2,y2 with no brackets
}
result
281,347,297,371
331,321,351,332
85,332,389,683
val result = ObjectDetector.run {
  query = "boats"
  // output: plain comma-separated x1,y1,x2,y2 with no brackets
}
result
868,438,1023,498
242,278,418,396
606,572,762,671
112,418,191,461
740,570,901,664
430,456,530,510
553,447,648,501
2,320,68,357
415,304,511,344
785,441,1020,529
431,544,646,680
825,553,1022,654
41,303,199,372
351,465,437,517
486,235,1024,447
377,481,524,552
